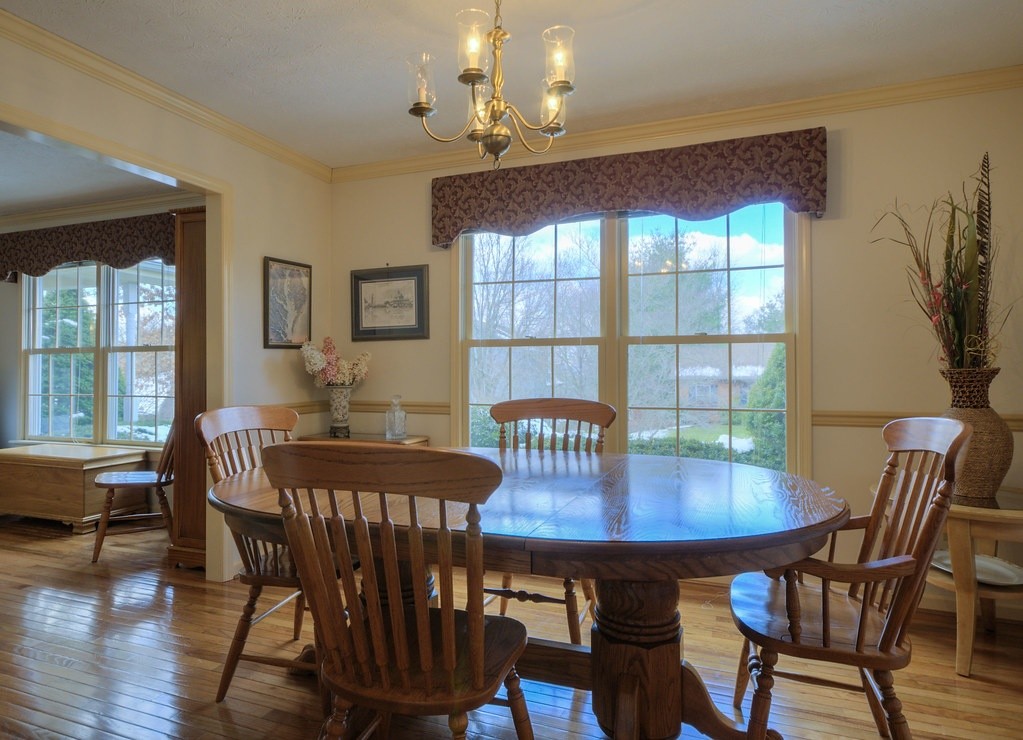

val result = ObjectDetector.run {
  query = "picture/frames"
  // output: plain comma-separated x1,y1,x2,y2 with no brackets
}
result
262,256,312,349
349,263,431,342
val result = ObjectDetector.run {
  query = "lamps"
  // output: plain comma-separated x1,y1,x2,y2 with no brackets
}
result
405,1,578,173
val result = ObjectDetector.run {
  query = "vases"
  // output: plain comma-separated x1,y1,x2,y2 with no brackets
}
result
939,366,1014,509
324,384,354,438
385,395,407,441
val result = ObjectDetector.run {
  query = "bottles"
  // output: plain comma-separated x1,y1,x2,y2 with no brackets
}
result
385,394,408,441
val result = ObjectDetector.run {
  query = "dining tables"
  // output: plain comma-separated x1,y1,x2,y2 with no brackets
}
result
206,445,850,740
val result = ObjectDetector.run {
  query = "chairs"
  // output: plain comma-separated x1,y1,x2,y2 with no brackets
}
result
485,399,617,647
257,438,535,739
192,405,360,704
91,417,178,564
728,417,974,740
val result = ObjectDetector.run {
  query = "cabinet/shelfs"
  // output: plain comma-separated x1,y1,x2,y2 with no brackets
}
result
0,443,149,534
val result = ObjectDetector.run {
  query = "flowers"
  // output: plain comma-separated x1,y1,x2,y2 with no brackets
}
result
300,336,371,389
917,270,991,367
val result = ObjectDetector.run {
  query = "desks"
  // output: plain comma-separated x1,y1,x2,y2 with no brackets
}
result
869,485,1023,678
298,431,430,448
